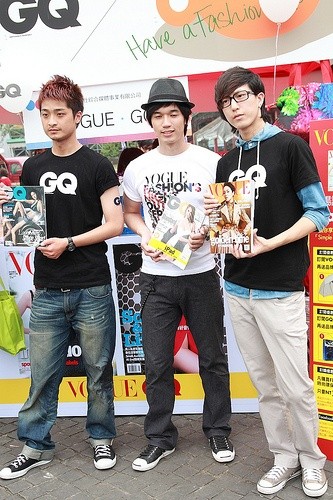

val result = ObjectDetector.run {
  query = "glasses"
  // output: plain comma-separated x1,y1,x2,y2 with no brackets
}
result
216,90,258,107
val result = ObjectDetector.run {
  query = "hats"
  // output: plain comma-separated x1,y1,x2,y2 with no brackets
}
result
141,79,194,110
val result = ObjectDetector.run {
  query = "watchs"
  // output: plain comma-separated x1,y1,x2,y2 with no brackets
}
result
66,237,76,252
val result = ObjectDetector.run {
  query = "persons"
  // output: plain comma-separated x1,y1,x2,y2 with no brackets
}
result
160,204,196,253
172,315,200,374
123,77,237,472
210,182,251,238
136,139,153,152
202,66,332,499
116,147,144,185
0,72,124,480
15,287,87,377
4,189,43,245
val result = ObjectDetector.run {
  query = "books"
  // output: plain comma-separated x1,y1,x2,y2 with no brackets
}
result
206,180,255,255
147,195,207,270
2,185,48,247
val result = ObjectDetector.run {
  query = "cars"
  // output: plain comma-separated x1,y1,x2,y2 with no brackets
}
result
1,154,12,187
4,156,29,183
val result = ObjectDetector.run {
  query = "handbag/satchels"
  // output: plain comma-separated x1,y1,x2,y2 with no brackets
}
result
0,276,26,356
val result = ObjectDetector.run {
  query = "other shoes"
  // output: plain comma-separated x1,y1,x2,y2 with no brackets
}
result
207,435,235,463
0,453,51,480
92,444,117,470
130,444,175,472
301,466,329,497
256,464,302,494
